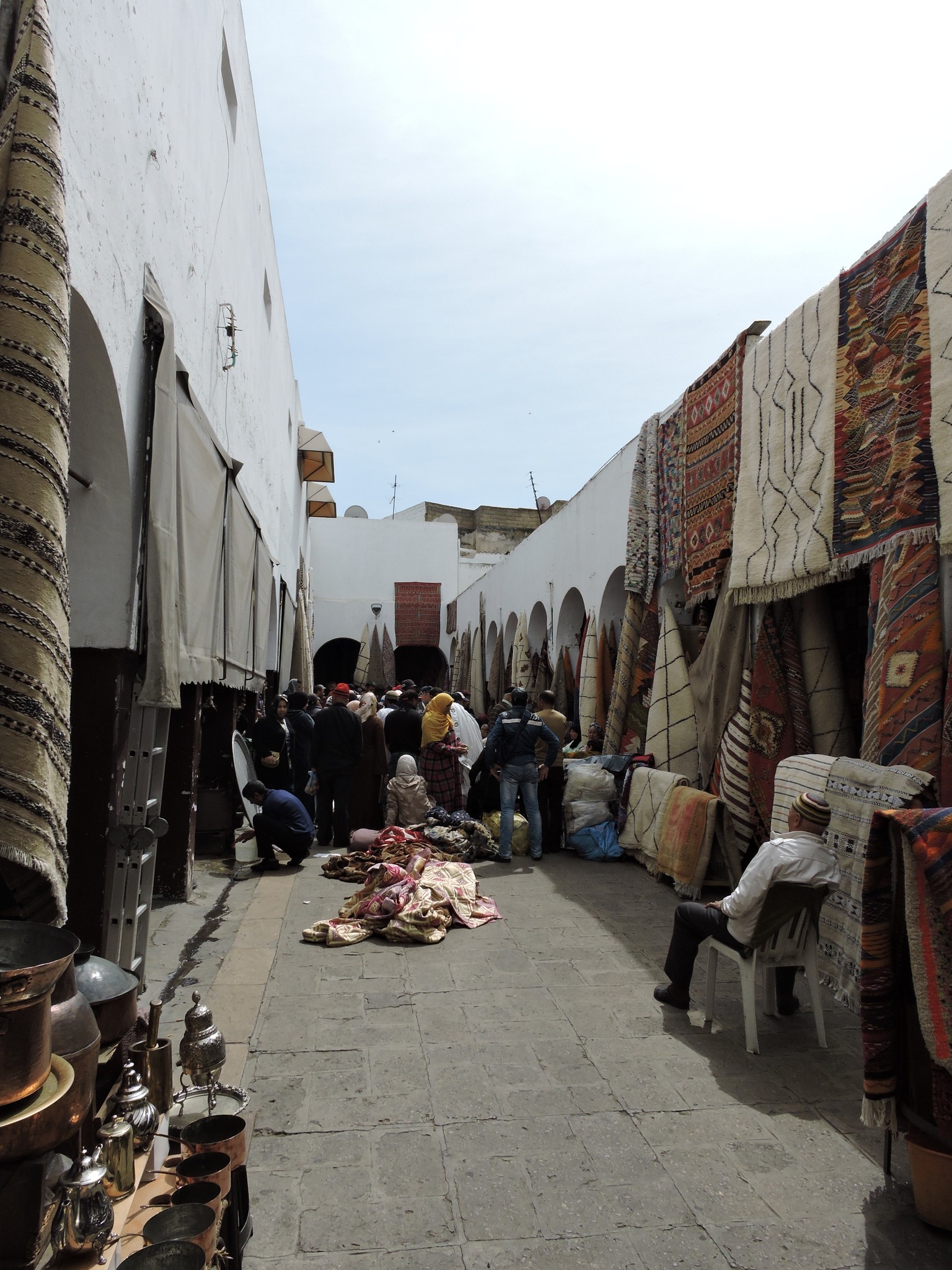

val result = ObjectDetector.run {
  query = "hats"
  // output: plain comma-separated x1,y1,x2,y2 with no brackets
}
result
401,679,417,691
332,683,350,699
317,684,326,689
420,686,433,695
288,691,309,709
308,693,320,704
504,686,515,695
326,696,332,705
385,690,399,700
792,792,831,826
402,689,418,702
392,685,402,691
451,692,470,702
511,688,528,700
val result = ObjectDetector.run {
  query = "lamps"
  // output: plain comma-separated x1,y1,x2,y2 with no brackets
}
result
371,604,382,619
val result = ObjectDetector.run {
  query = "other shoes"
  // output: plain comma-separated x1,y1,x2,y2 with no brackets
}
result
287,849,311,866
531,854,543,861
778,994,799,1014
550,843,560,853
654,986,690,1010
542,844,550,854
250,859,280,872
489,852,511,863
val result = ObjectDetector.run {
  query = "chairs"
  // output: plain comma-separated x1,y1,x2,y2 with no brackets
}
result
703,881,828,1055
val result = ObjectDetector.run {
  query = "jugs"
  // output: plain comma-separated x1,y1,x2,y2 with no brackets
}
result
94,1115,135,1203
108,1059,159,1152
45,1143,115,1270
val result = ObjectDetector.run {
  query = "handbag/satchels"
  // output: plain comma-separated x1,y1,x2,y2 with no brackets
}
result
260,751,280,768
378,774,388,805
304,771,319,796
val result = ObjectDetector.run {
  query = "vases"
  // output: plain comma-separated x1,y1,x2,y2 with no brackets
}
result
903,1131,952,1231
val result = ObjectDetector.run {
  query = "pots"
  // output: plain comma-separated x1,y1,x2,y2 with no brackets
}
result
0,920,81,1005
145,1115,247,1172
115,1239,209,1270
146,1151,231,1199
0,953,101,1163
140,1181,221,1225
72,945,140,1046
96,1204,217,1265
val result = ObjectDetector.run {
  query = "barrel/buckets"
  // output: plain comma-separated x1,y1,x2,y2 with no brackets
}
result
234,828,259,862
903,1134,952,1233
0,983,56,1106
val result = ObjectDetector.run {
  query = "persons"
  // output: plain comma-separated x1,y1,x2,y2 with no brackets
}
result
235,679,605,871
654,792,841,1015
